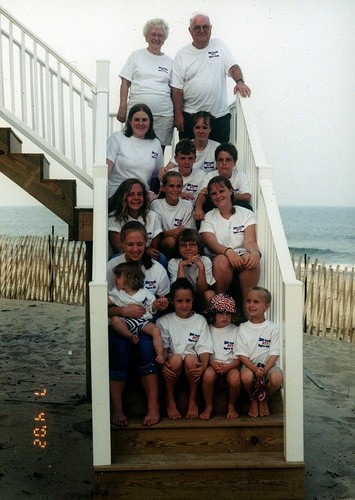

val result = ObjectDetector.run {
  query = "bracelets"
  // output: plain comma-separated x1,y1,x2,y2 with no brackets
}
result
253,249,262,259
237,78,245,83
224,247,232,254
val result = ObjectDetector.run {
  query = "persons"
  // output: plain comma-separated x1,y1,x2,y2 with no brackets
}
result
170,14,251,144
106,103,165,211
107,178,284,427
117,16,174,151
157,110,254,231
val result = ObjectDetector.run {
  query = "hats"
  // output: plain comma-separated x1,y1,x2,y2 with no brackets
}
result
202,292,235,314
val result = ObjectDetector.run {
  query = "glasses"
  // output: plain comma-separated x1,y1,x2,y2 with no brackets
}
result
179,243,197,247
